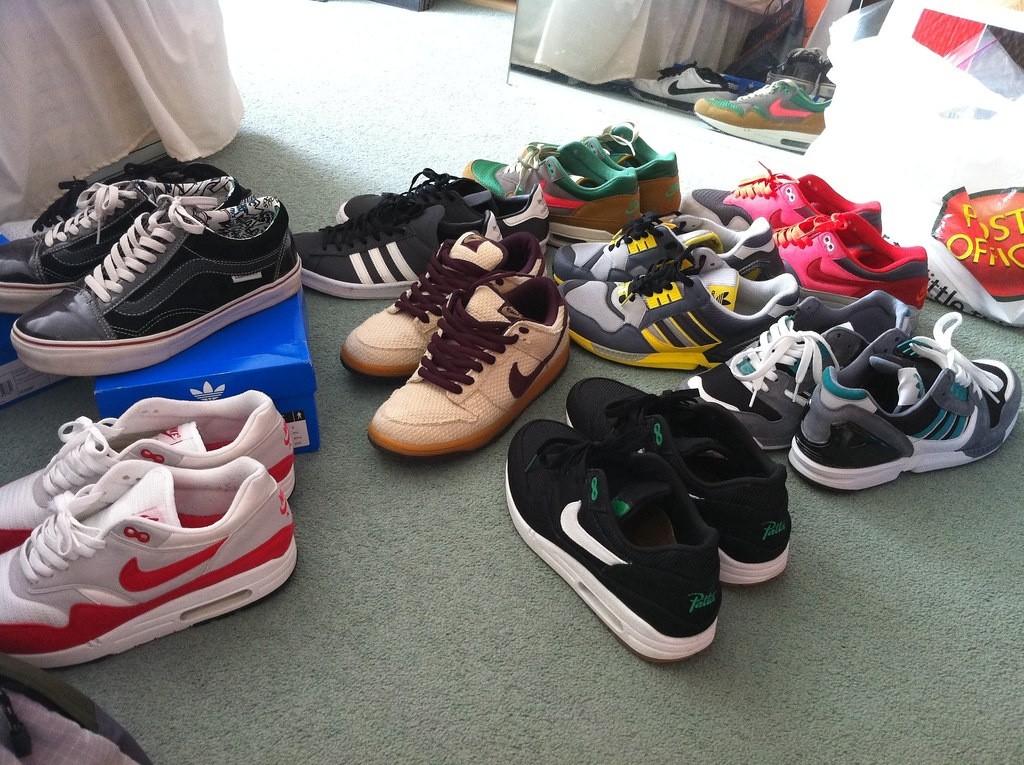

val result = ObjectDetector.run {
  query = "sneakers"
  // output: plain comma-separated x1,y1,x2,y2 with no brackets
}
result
0,173,255,318
695,79,832,153
11,194,302,377
0,388,295,551
504,417,725,662
766,44,836,100
463,141,645,245
674,290,920,451
631,63,743,112
561,378,794,586
0,456,297,668
339,232,549,377
334,176,549,253
790,311,1024,487
367,275,571,461
290,191,502,299
547,161,930,369
524,126,683,217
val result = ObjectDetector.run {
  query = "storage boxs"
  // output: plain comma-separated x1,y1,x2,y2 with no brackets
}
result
0,218,75,411
91,278,324,459
